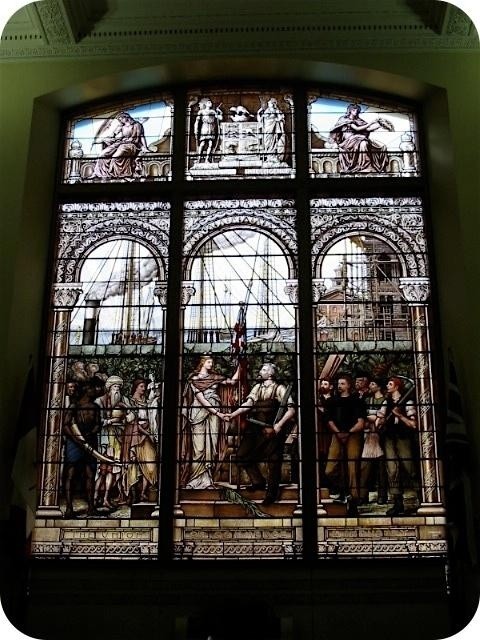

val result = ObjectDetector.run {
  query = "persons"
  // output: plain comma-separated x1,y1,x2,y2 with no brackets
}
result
328,104,387,174
60,357,421,520
192,97,222,162
91,111,144,179
257,98,285,163
